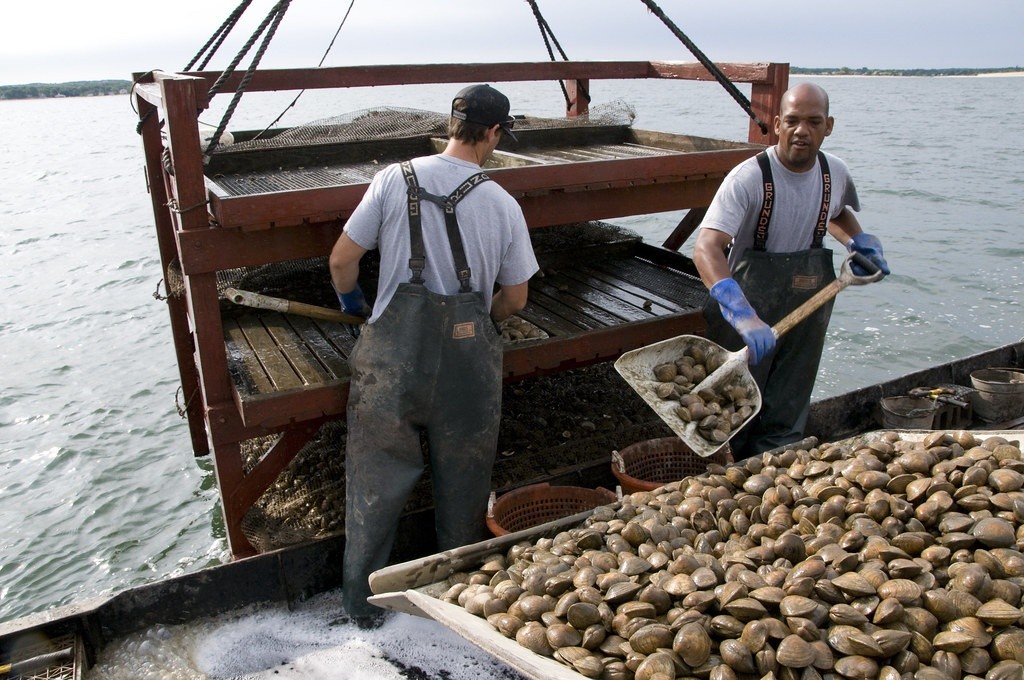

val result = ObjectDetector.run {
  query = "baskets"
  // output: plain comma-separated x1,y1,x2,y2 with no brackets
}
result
612,436,735,496
487,481,620,536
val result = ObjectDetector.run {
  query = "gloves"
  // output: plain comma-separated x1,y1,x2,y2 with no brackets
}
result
710,278,776,367
335,283,370,338
846,233,890,280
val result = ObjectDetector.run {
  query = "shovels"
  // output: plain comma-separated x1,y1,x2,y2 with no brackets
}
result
226,285,369,329
612,252,884,460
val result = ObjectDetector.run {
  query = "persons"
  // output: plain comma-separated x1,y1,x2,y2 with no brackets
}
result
692,81,890,471
327,83,542,628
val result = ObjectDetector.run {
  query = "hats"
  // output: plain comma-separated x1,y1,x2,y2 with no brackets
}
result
452,84,521,143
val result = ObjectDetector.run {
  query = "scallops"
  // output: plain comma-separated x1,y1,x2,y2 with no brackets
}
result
485,311,552,344
651,345,758,444
439,429,1024,680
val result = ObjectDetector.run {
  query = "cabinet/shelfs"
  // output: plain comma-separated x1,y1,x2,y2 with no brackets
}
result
132,60,792,562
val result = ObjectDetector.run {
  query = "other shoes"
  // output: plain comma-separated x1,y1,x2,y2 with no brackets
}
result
351,608,387,630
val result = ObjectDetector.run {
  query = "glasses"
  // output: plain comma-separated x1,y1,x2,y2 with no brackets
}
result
496,114,515,129
